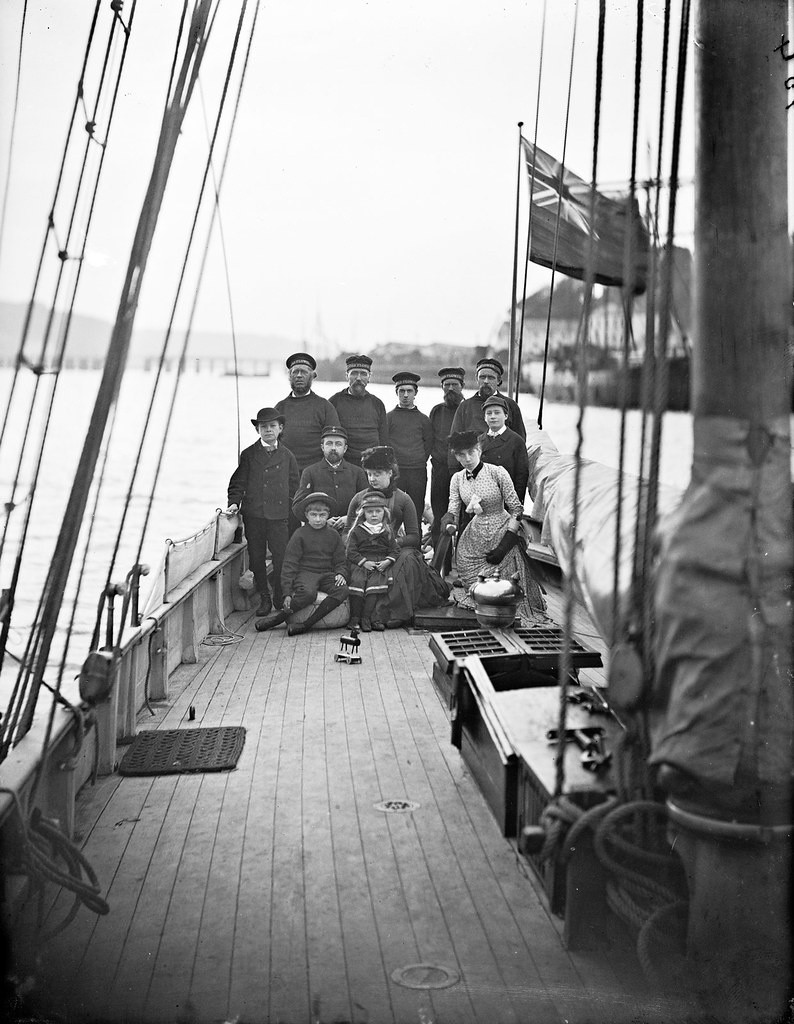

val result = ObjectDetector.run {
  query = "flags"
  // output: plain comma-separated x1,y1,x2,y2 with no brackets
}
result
521,135,649,295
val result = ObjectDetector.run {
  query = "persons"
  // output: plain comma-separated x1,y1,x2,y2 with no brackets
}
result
329,355,386,466
254,491,350,636
274,353,341,477
430,366,467,575
346,445,448,629
447,359,527,527
446,431,548,628
387,371,430,552
346,491,401,634
478,396,529,517
227,408,300,616
291,425,369,536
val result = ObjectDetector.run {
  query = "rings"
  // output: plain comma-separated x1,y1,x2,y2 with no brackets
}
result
340,526,342,528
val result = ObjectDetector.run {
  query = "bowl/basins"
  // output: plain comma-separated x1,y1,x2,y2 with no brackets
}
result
475,605,518,628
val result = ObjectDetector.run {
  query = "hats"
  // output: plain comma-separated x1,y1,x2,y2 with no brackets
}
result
360,446,397,470
447,430,477,451
251,408,285,426
475,358,504,376
296,492,337,522
286,353,316,370
346,355,372,373
392,371,421,388
438,367,465,383
321,426,348,440
362,491,386,507
481,396,509,413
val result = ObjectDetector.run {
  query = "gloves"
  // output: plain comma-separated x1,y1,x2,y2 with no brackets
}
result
440,513,455,537
486,530,519,565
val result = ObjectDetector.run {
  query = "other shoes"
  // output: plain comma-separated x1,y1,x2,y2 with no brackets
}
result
255,616,281,631
386,619,402,628
256,597,272,616
361,617,371,632
272,591,284,611
347,617,360,630
287,623,306,636
371,621,385,631
